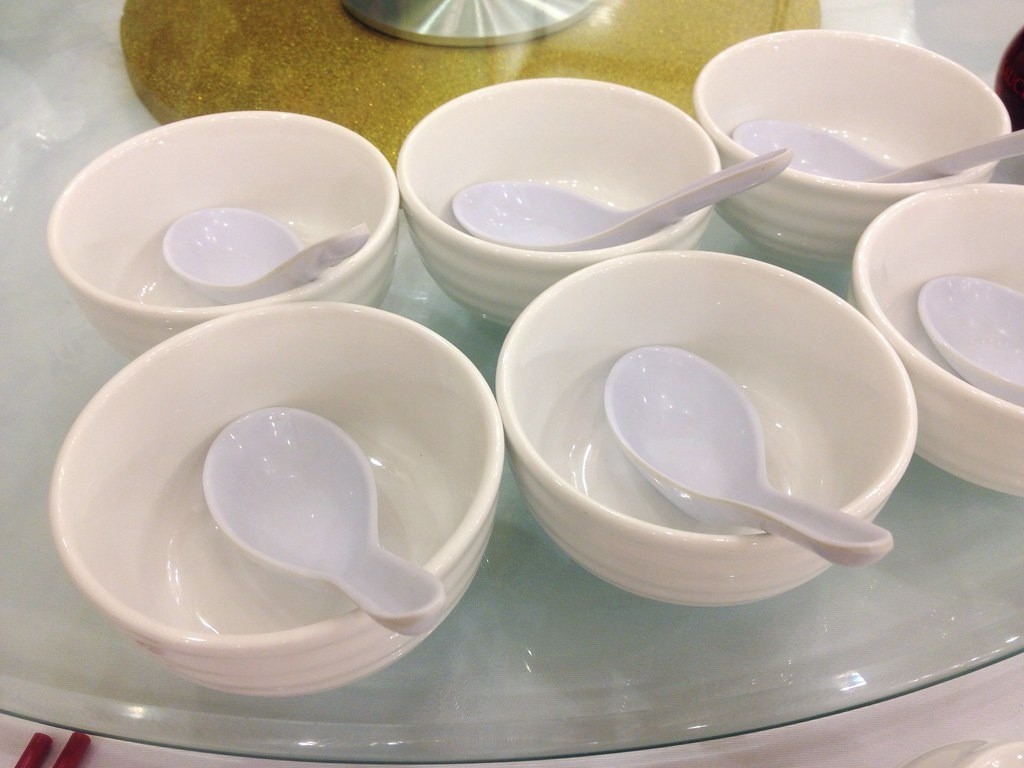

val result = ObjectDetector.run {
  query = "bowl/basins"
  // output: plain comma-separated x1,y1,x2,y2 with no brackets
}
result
395,75,724,331
496,250,918,609
47,303,503,699
694,30,1011,262
843,182,1024,498
45,109,398,369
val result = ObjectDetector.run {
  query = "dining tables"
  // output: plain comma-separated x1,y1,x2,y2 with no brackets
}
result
0,0,1023,768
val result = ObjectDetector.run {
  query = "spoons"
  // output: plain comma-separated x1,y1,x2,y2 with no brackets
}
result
203,407,448,634
919,274,1024,407
602,346,894,569
158,201,369,304
731,119,1024,183
449,150,800,249
